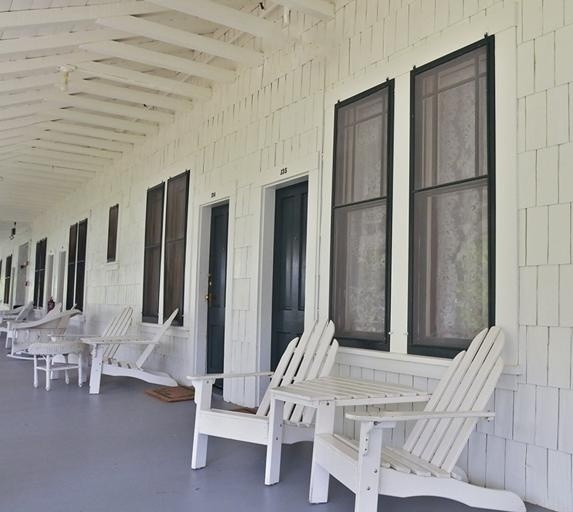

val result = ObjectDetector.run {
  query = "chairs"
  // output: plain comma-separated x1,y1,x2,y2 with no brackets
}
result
184,311,341,490
307,319,539,511
0,283,180,397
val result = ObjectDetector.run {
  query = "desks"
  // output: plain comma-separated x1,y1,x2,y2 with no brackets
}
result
259,365,435,507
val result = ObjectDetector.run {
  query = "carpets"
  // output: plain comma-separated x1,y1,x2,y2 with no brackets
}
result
141,384,196,404
